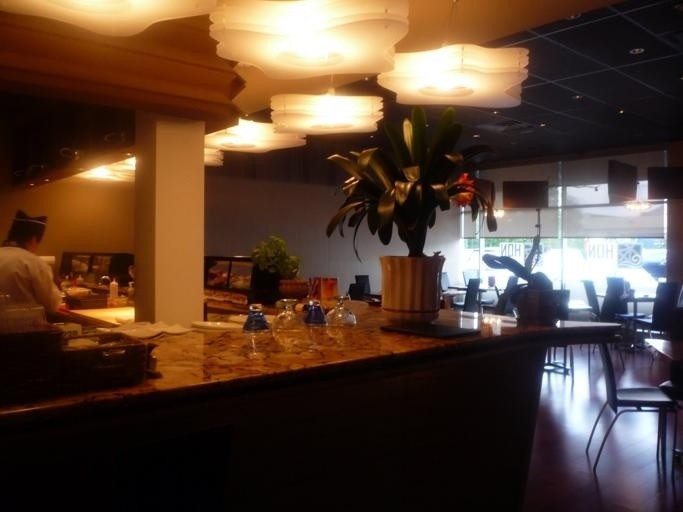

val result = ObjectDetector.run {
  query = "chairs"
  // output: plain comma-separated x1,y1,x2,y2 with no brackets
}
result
439,267,682,373
585,341,674,475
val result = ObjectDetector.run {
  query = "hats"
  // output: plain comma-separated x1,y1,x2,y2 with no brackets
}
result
14,210,48,225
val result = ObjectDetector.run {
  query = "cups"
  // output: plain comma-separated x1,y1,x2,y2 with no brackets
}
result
305,300,325,324
242,304,268,331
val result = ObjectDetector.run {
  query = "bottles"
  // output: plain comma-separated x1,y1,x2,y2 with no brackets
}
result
109,278,117,300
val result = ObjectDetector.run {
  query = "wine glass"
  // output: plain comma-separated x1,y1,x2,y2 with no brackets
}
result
325,295,357,326
273,299,305,332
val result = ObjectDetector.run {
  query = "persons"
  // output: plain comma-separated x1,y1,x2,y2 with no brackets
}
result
0,210,61,324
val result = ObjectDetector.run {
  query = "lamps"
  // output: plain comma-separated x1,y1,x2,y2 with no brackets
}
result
377,44,529,108
203,120,306,153
208,1,410,81
203,148,224,167
270,94,383,133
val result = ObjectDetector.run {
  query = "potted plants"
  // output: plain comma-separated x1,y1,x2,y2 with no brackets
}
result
483,234,570,327
252,230,312,299
318,102,502,332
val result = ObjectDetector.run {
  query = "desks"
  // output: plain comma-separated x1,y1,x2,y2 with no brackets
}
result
644,338,683,466
